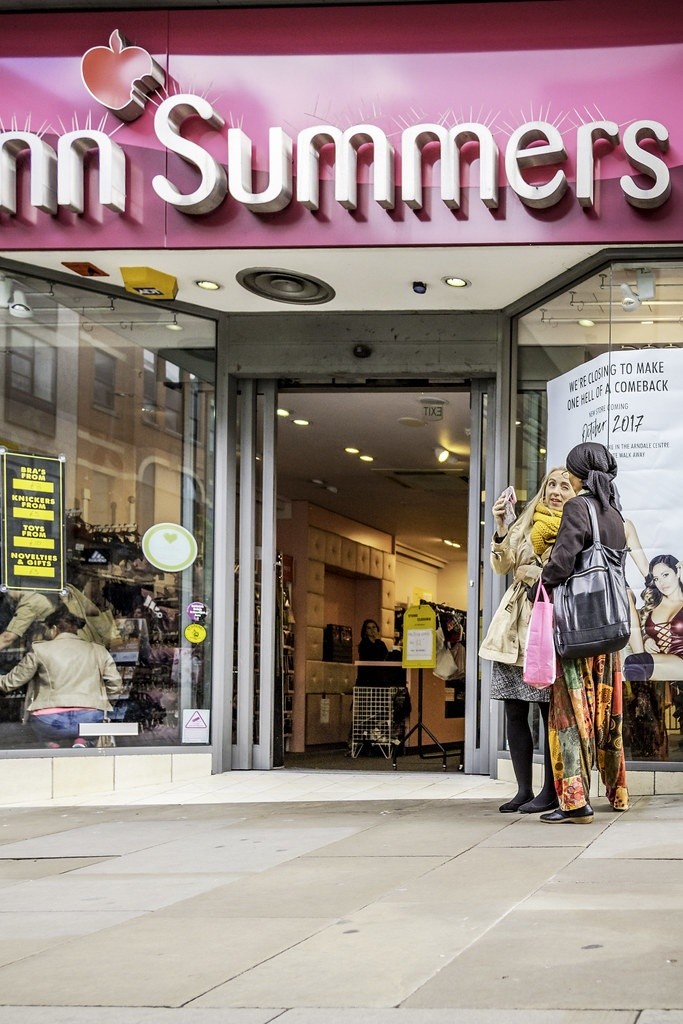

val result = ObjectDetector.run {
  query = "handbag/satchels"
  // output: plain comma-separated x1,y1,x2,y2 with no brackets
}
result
97,686,116,748
523,573,556,690
552,496,632,658
67,585,122,647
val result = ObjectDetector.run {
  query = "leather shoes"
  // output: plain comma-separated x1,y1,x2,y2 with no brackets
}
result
540,803,594,823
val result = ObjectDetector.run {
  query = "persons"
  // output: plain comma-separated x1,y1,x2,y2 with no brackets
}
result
0,577,154,749
478,467,574,811
523,444,628,823
357,619,389,687
619,554,683,747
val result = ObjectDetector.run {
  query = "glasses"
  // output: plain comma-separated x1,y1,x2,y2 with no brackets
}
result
562,470,570,479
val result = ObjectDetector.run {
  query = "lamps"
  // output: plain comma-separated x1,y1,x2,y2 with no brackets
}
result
8,289,34,318
620,282,641,312
434,447,449,464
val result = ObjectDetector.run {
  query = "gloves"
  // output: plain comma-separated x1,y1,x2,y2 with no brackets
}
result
492,498,508,537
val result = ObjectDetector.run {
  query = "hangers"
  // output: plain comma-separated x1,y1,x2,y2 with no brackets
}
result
108,575,128,585
64,507,142,538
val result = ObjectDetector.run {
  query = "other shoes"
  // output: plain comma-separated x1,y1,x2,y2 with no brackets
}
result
44,740,64,749
72,738,94,748
499,793,534,813
518,797,558,813
